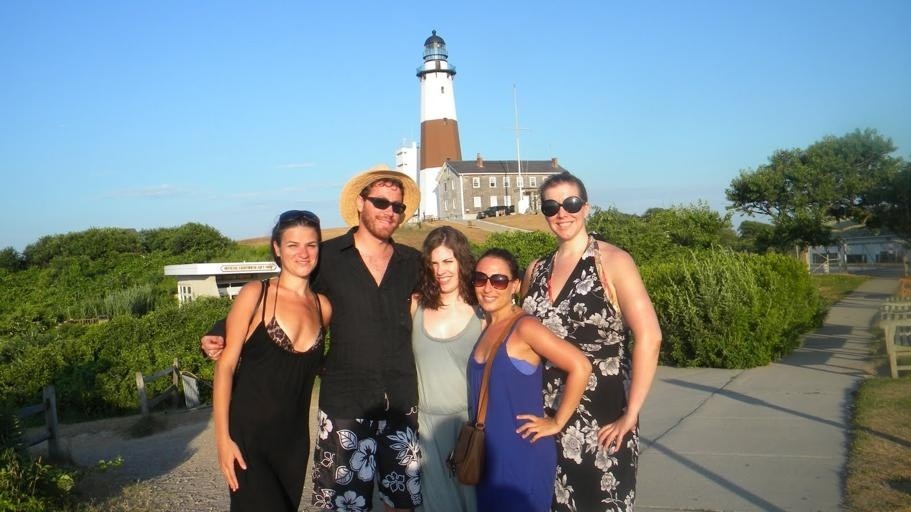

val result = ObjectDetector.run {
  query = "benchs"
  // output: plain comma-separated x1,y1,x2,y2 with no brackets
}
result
879,296,911,380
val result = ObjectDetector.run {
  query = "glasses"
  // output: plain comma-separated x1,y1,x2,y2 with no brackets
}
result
471,272,513,290
542,197,584,217
365,196,406,214
277,210,321,238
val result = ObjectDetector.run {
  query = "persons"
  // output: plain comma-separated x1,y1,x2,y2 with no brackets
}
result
198,162,432,512
520,170,664,511
406,224,525,512
465,246,594,511
210,209,332,510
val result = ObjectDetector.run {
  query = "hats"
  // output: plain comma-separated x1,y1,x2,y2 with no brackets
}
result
339,165,421,227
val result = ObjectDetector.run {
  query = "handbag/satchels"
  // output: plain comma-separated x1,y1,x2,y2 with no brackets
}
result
446,423,486,485
